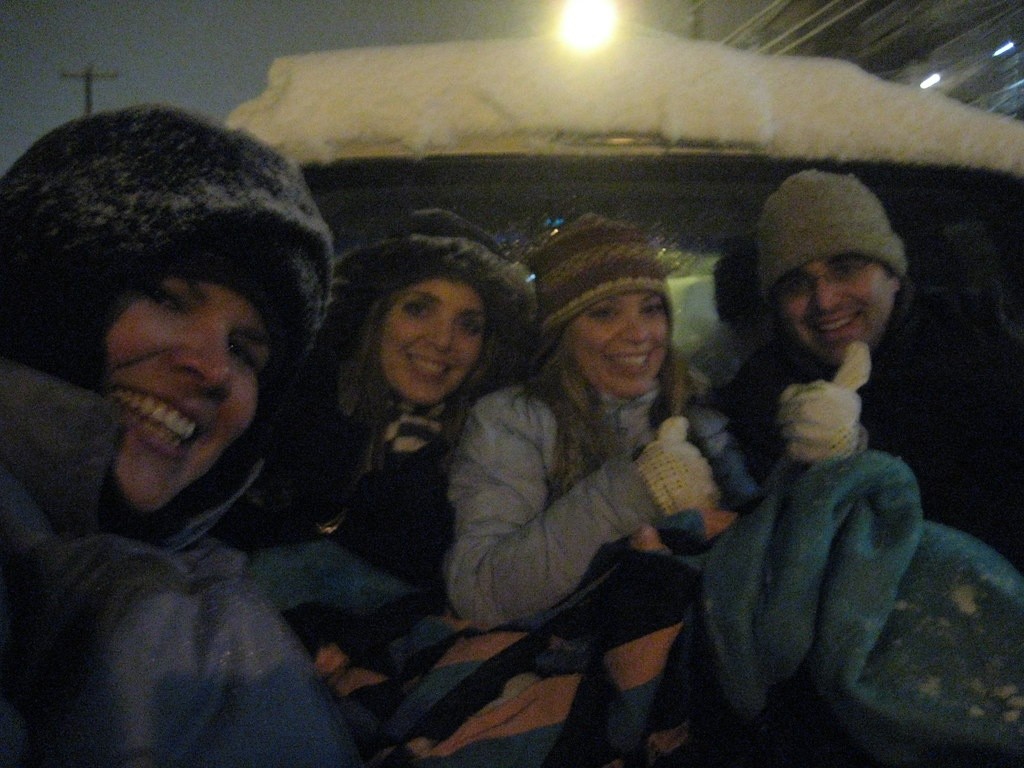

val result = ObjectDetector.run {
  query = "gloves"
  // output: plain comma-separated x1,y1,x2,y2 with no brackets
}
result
635,417,714,513
779,344,871,460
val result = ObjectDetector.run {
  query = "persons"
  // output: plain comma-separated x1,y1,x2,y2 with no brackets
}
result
436,219,873,766
0,102,357,768
204,204,534,707
690,162,1024,768
681,227,776,384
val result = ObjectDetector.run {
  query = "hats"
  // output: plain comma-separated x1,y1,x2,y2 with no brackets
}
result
528,214,673,336
757,170,907,290
337,211,532,352
0,111,328,393
